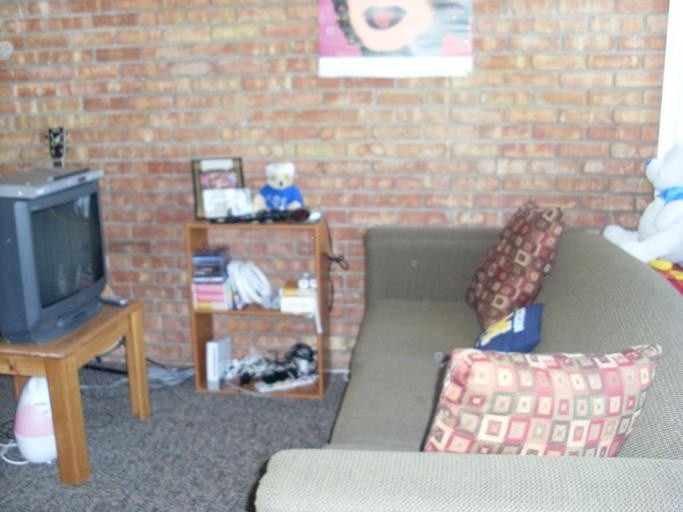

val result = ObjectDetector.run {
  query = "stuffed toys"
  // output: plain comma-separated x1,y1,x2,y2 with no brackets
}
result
253,162,303,211
602,140,682,267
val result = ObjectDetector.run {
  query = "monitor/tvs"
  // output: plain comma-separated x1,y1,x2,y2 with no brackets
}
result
0,178,107,344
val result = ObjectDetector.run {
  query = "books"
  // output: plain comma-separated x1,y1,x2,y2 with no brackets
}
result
192,244,317,315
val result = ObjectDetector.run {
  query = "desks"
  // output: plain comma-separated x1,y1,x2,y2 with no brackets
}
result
0,301,151,484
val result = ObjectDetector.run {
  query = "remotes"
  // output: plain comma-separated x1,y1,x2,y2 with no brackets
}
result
100,294,129,307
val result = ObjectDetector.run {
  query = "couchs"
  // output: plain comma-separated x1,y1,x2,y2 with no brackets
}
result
254,213,683,512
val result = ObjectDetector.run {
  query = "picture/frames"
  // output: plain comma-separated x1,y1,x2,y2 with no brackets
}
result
190,156,243,221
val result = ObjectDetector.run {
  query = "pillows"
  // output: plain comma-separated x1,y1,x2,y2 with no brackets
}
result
464,195,565,330
424,341,663,465
476,297,545,353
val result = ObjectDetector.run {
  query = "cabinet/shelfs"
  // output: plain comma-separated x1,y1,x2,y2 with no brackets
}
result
187,218,328,400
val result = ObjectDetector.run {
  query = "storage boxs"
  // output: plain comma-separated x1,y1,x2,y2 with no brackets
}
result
278,276,317,316
191,246,230,283
192,278,233,314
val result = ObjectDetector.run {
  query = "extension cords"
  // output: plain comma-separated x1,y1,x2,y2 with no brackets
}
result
253,370,314,394
